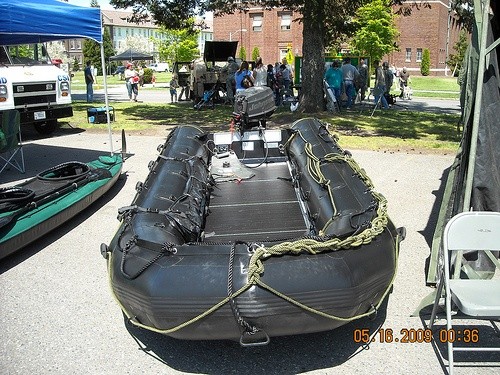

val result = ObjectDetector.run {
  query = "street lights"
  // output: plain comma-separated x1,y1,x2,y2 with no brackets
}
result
229,29,247,42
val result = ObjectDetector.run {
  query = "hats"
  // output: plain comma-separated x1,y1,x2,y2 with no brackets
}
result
127,63,134,67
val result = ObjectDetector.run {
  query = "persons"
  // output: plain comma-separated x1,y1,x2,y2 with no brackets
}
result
399,66,411,87
170,63,190,102
138,67,145,87
373,60,394,111
125,63,140,102
323,56,369,110
85,60,94,103
221,57,293,105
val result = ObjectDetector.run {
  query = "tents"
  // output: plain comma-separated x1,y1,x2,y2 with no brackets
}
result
0,0,113,158
108,47,153,63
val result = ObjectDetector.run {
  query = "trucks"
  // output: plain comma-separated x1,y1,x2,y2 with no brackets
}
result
0,43,73,135
148,63,169,73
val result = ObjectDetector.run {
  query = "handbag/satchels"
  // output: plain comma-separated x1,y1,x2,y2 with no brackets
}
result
241,75,254,88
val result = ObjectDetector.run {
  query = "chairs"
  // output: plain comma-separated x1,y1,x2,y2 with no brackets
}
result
359,88,386,118
0,110,25,174
428,211,500,375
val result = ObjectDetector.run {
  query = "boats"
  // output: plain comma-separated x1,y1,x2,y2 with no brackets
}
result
101,86,408,347
0,128,127,259
